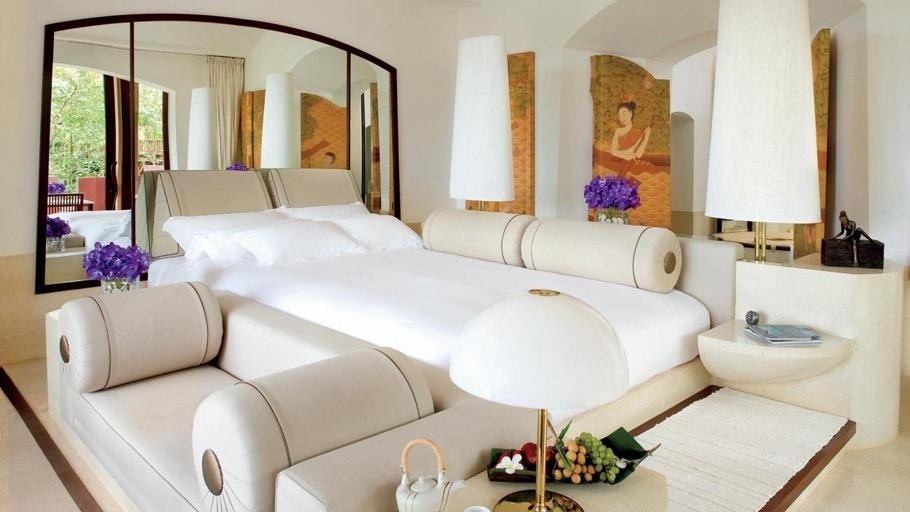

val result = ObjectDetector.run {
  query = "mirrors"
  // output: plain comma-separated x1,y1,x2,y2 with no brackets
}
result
33,12,402,295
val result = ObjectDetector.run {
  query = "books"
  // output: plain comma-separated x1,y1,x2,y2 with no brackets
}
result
744,323,826,343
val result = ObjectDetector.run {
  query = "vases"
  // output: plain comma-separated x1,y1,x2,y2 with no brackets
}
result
45,236,64,252
595,206,630,224
99,278,136,295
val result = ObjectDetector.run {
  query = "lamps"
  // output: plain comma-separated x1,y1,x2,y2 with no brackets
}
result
446,34,516,212
260,71,302,169
701,0,824,266
187,84,218,170
446,287,633,512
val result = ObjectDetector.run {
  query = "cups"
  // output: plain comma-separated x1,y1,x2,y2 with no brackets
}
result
465,506,491,512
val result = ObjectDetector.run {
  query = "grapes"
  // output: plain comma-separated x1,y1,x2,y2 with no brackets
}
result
576,432,619,484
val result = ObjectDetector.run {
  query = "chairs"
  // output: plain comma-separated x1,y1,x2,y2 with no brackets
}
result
47,192,84,214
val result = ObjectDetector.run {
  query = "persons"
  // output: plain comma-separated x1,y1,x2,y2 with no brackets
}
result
828,209,876,268
610,100,656,168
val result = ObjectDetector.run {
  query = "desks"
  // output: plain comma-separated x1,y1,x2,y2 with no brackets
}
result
443,385,863,511
47,199,95,211
697,250,907,450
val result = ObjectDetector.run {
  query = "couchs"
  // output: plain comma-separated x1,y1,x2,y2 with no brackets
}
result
43,279,551,512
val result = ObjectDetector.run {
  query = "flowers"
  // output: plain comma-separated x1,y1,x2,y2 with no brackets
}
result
582,174,642,223
225,162,249,172
46,214,72,238
47,181,66,194
79,240,153,293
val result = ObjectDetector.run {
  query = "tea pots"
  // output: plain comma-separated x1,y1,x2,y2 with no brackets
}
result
395,436,469,512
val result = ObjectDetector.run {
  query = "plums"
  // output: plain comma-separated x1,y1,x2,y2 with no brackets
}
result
511,450,521,459
498,452,510,463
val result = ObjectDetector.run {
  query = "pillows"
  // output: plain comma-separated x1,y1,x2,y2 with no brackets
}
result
520,215,684,294
52,280,232,398
189,343,438,512
162,199,424,283
419,207,537,268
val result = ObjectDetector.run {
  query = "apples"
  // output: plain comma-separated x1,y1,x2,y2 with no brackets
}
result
522,443,553,464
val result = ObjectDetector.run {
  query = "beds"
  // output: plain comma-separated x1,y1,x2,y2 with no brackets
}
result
46,168,164,281
147,167,745,450
